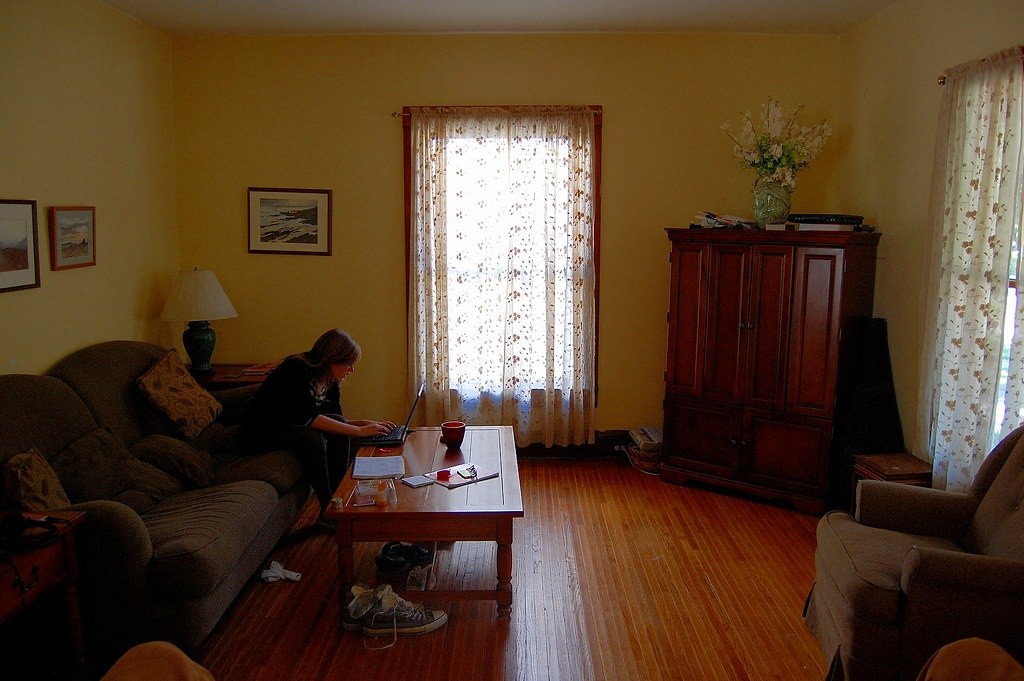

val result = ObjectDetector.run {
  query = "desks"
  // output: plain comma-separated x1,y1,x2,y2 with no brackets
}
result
1,504,89,680
180,362,279,391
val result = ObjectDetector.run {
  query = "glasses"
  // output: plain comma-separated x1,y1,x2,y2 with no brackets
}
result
337,358,356,368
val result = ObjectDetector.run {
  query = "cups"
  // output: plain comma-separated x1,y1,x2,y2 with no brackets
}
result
441,421,465,450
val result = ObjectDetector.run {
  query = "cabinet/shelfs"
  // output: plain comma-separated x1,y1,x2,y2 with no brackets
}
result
658,227,883,514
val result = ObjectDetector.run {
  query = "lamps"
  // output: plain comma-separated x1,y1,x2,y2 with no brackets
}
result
158,266,239,377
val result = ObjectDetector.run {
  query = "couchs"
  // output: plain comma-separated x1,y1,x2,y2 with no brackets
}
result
1,341,316,653
798,424,1024,681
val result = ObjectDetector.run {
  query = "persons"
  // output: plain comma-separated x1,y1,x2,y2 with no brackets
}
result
244,327,397,529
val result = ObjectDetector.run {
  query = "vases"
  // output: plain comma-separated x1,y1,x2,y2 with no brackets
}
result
751,177,791,229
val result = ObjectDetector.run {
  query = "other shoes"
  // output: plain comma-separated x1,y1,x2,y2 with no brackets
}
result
316,508,338,528
375,553,436,579
382,541,437,567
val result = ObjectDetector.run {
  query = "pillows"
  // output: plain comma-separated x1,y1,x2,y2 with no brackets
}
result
45,423,183,517
5,447,69,512
135,347,222,441
131,435,217,488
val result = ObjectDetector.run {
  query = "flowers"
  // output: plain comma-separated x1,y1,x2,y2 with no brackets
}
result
720,92,832,191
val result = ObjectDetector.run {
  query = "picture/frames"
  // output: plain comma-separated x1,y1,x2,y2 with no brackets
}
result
47,205,97,271
0,199,41,294
247,186,333,257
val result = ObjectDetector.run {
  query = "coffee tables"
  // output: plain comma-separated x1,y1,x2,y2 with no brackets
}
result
325,425,522,619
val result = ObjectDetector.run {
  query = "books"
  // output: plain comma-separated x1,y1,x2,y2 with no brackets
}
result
627,427,663,449
352,456,405,479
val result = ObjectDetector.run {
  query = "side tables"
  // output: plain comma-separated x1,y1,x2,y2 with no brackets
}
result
849,452,933,518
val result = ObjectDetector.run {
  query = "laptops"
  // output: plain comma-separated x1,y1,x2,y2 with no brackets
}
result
354,383,424,443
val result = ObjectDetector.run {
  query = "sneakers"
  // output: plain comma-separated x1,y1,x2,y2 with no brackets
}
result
343,584,424,629
363,587,448,651
